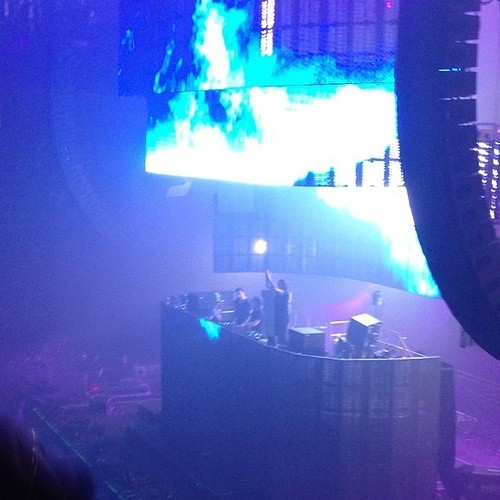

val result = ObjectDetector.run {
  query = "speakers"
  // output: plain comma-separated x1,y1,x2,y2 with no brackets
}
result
186,291,215,320
289,325,326,358
346,313,383,348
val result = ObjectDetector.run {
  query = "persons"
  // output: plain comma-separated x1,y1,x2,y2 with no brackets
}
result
231,288,250,327
264,269,291,345
239,297,263,333
